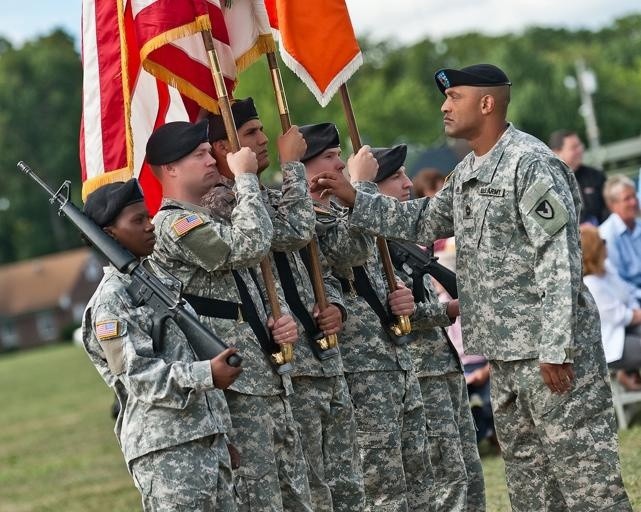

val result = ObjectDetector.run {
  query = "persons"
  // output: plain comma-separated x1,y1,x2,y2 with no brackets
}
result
82,63,641,512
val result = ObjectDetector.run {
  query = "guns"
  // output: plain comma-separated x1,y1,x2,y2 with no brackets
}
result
16,159,243,368
386,236,458,303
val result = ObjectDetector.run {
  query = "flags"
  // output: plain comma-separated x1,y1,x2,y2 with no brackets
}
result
79,1,363,220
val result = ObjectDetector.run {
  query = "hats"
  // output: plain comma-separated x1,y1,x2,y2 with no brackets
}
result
144,120,211,167
79,178,146,234
433,63,512,101
210,95,258,141
294,121,341,162
367,142,407,179
